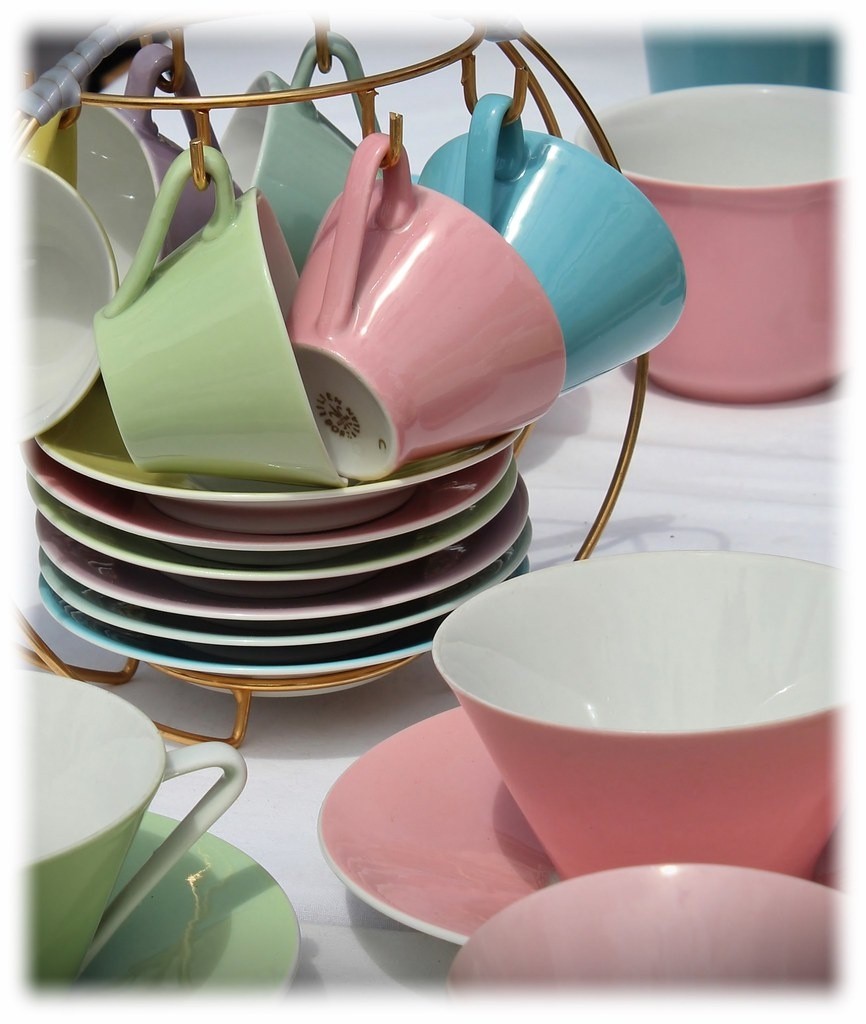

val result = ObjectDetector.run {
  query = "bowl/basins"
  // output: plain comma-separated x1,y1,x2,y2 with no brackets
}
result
430,550,843,880
448,863,841,996
571,81,837,404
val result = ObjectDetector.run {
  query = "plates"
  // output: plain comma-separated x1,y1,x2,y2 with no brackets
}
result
34,515,534,658
31,474,530,630
25,455,520,603
31,371,527,536
23,438,522,569
314,701,574,953
35,557,535,701
72,808,305,997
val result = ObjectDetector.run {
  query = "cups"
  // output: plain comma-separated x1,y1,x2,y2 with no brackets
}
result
217,34,388,278
289,132,566,481
17,666,251,997
410,91,687,399
18,103,118,449
90,149,350,494
72,41,242,292
